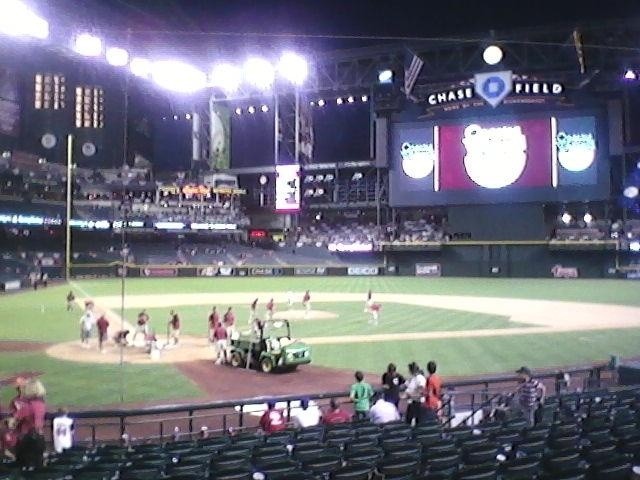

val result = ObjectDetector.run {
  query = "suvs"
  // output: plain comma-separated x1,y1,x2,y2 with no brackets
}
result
225,311,311,375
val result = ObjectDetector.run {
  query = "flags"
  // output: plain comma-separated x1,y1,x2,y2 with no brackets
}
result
403,46,424,94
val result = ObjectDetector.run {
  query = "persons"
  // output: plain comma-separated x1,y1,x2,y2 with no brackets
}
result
223,307,235,336
66,290,181,354
364,288,373,312
371,303,382,319
302,290,310,312
0,381,75,466
214,322,228,364
266,299,274,320
208,307,219,339
259,362,442,434
515,367,547,426
